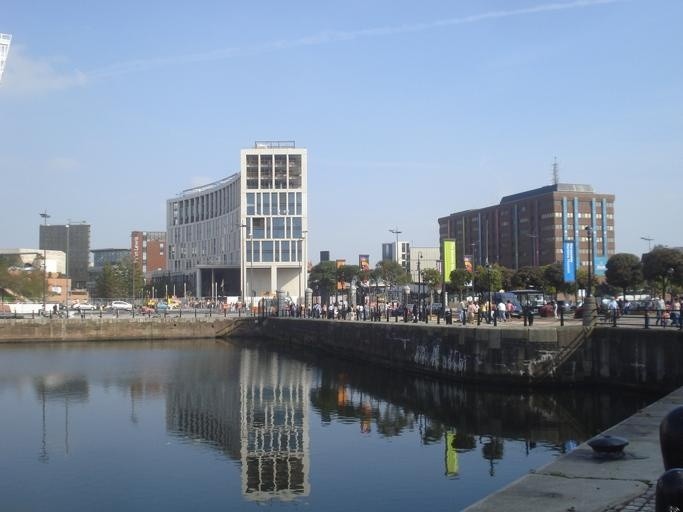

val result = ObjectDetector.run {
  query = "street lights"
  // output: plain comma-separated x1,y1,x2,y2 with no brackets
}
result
470,241,475,298
129,256,140,312
129,386,140,425
38,381,50,466
63,219,70,309
583,224,592,297
416,250,423,304
388,228,402,263
484,263,492,323
136,278,218,307
639,235,653,251
38,207,50,311
63,393,72,459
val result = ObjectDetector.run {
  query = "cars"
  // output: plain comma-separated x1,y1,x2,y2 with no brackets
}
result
71,302,97,310
490,289,657,318
140,298,255,315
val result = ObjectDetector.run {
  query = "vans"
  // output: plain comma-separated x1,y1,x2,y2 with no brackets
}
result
259,295,295,311
107,301,132,311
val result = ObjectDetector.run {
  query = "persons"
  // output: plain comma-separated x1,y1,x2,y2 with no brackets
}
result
40,304,44,315
183,295,681,326
52,304,57,315
